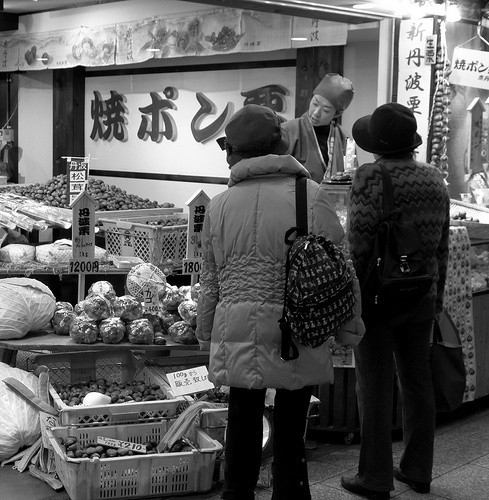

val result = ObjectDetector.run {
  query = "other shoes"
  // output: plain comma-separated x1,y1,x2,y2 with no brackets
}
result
392,464,430,492
340,474,392,499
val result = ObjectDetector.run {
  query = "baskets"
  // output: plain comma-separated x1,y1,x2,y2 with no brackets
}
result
98,214,188,268
26,350,228,500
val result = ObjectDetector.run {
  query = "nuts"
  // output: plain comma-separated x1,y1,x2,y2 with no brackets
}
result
88,177,188,226
54,378,228,487
0,175,67,208
451,211,479,223
330,172,352,180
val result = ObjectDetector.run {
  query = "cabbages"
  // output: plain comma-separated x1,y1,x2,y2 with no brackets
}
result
0,361,50,459
0,277,57,338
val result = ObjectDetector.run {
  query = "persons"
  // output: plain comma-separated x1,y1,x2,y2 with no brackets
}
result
346,102,450,500
195,104,366,500
280,73,355,183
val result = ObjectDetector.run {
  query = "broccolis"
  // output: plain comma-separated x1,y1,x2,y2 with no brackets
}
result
51,280,200,345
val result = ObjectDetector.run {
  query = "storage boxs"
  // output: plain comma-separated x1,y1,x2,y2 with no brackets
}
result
98,212,189,267
25,348,321,500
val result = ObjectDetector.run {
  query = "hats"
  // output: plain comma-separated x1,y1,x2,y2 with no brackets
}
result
313,72,356,116
352,103,422,155
215,104,287,165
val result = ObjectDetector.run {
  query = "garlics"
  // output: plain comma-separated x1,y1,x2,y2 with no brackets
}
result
431,49,451,180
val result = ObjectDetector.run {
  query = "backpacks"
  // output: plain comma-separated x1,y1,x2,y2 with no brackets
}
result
278,174,356,359
365,165,432,308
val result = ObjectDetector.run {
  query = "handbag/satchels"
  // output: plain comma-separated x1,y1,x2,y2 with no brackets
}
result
429,307,466,410
